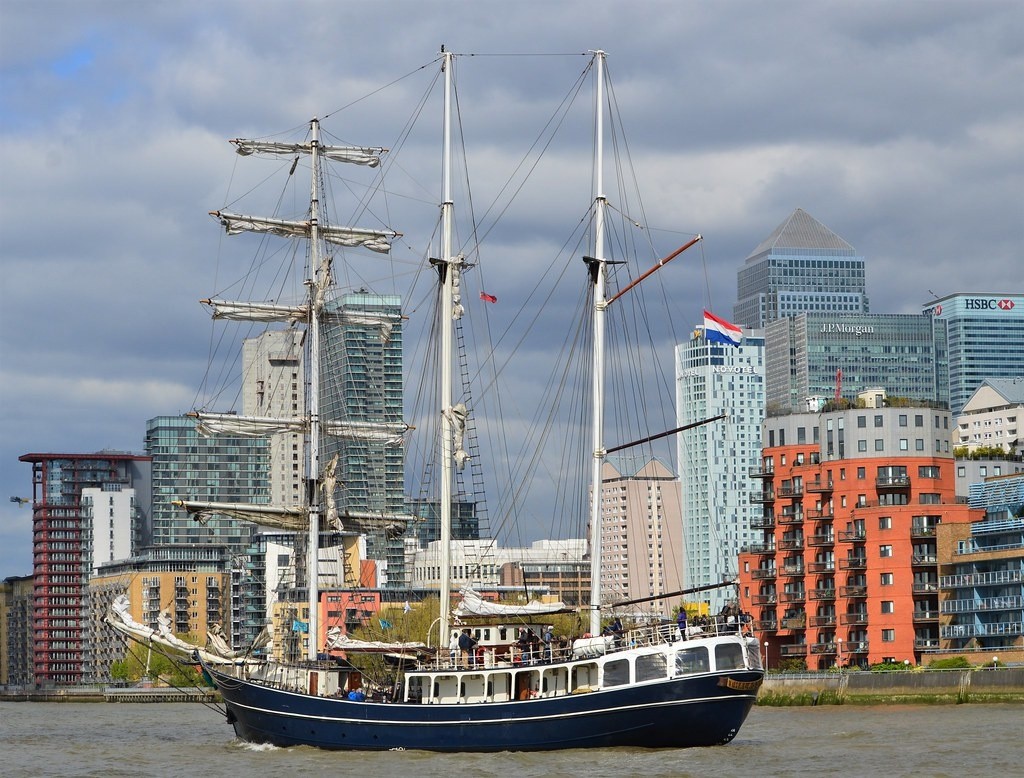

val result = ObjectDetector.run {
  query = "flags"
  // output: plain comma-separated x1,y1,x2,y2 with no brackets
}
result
294,621,307,633
480,291,497,304
705,310,741,349
379,618,393,629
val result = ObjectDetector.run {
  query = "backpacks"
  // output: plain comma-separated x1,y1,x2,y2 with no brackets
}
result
547,632,554,639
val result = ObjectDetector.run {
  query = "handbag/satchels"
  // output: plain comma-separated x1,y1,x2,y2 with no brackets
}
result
613,633,621,640
727,615,735,627
472,644,479,648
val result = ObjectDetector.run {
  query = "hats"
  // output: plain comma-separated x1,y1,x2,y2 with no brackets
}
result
548,626,553,629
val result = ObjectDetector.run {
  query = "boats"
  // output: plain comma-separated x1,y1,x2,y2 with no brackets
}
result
99,40,768,754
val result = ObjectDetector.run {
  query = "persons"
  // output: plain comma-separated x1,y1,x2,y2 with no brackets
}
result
450,601,754,670
336,685,394,704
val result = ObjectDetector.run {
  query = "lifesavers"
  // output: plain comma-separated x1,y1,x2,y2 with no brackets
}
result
476,647,489,662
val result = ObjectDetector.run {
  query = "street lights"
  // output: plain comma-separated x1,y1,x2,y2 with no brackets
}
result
903,660,909,672
992,656,999,670
838,638,843,673
763,642,770,673
